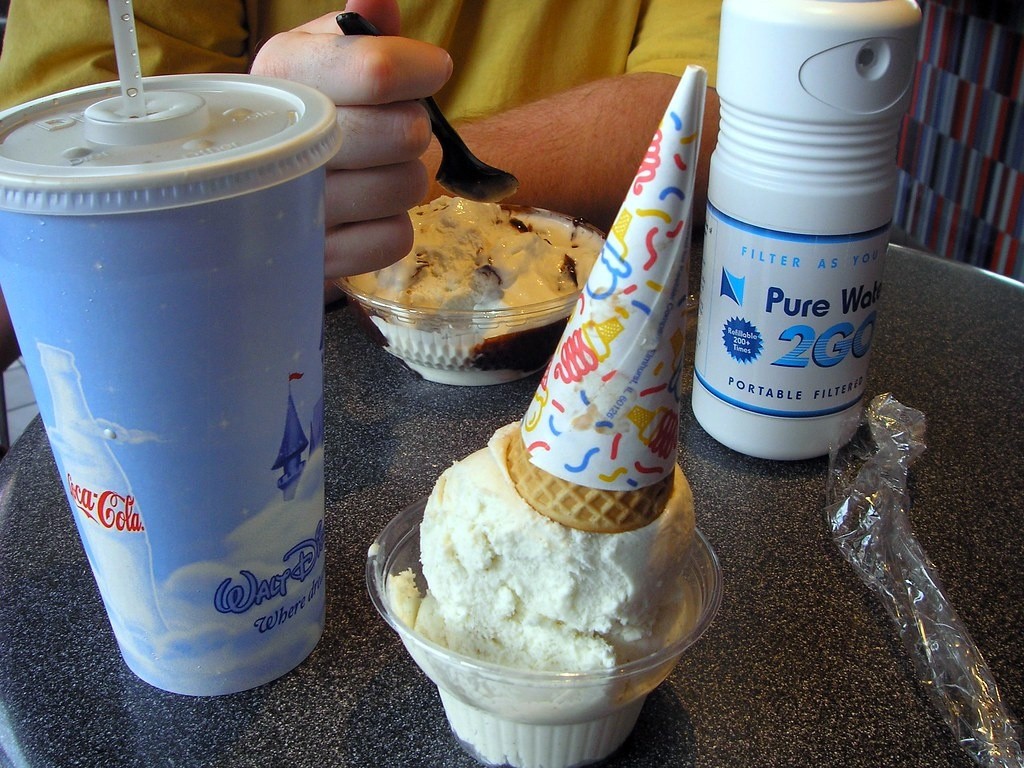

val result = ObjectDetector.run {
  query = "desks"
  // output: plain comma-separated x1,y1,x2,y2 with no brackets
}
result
0,221,1024,768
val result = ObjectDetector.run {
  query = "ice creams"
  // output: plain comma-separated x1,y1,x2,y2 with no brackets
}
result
341,191,610,386
382,419,698,768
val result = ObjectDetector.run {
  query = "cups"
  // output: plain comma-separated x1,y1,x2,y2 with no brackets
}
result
0,73,347,696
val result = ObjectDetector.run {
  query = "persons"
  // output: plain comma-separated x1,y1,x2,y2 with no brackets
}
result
0,0,723,346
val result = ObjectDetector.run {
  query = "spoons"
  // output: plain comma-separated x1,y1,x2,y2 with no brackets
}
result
337,13,519,202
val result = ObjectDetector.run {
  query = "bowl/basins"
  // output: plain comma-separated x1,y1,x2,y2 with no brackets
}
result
328,205,607,389
366,497,725,768
